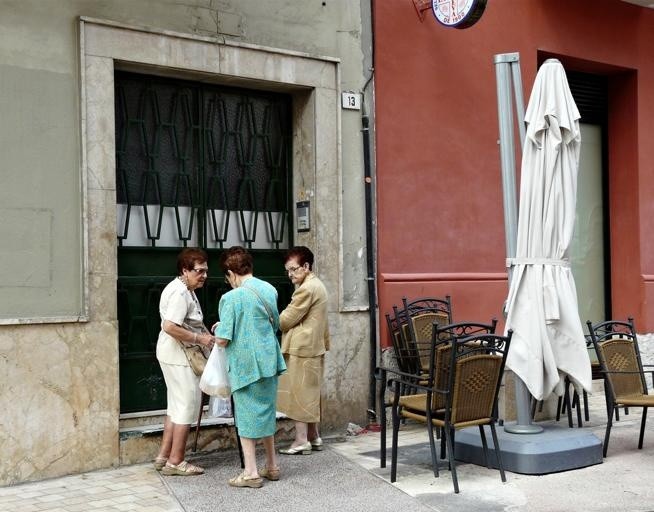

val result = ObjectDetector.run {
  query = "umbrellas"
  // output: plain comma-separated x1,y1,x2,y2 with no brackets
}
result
505,57,591,405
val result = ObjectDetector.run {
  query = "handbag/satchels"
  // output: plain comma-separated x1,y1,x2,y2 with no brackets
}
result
173,317,213,377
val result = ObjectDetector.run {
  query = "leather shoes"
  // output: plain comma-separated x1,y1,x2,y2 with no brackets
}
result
259,464,281,481
228,470,263,489
154,457,204,476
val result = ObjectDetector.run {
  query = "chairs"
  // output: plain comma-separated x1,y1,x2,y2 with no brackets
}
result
374,295,513,494
586,315,654,457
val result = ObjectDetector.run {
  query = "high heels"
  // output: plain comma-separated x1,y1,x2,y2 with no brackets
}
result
278,437,323,454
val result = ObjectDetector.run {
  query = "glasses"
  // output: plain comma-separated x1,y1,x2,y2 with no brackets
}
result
194,268,209,274
287,265,300,274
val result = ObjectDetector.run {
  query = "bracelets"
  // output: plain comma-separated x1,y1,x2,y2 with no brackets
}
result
193,333,197,344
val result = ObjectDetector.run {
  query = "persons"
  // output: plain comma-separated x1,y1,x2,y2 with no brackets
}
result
213,246,289,488
155,248,216,476
274,246,329,456
